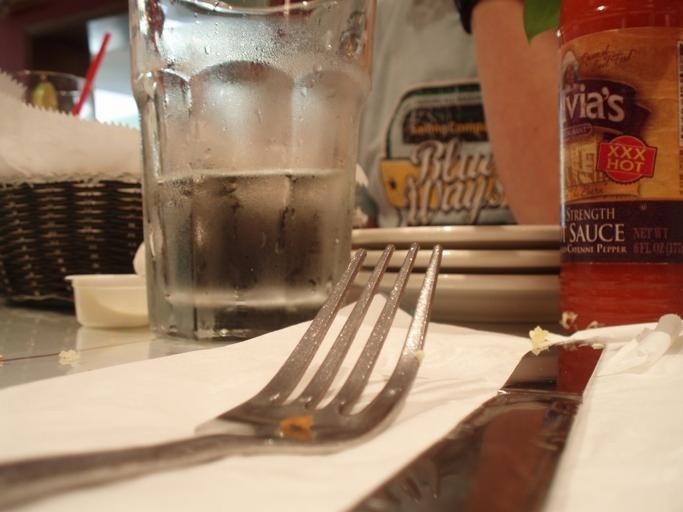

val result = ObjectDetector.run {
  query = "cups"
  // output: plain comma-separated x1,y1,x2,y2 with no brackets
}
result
126,0,376,340
7,71,96,123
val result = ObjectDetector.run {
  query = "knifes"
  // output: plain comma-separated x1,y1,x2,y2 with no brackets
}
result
349,336,604,511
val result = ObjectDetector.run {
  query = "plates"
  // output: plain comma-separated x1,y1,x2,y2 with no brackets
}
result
352,222,564,325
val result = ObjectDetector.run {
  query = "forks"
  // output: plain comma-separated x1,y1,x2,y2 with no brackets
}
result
1,243,443,512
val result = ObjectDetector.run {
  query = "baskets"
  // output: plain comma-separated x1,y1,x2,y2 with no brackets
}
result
0,177,144,311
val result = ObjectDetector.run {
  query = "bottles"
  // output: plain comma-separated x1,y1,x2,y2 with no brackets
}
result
557,1,683,333
